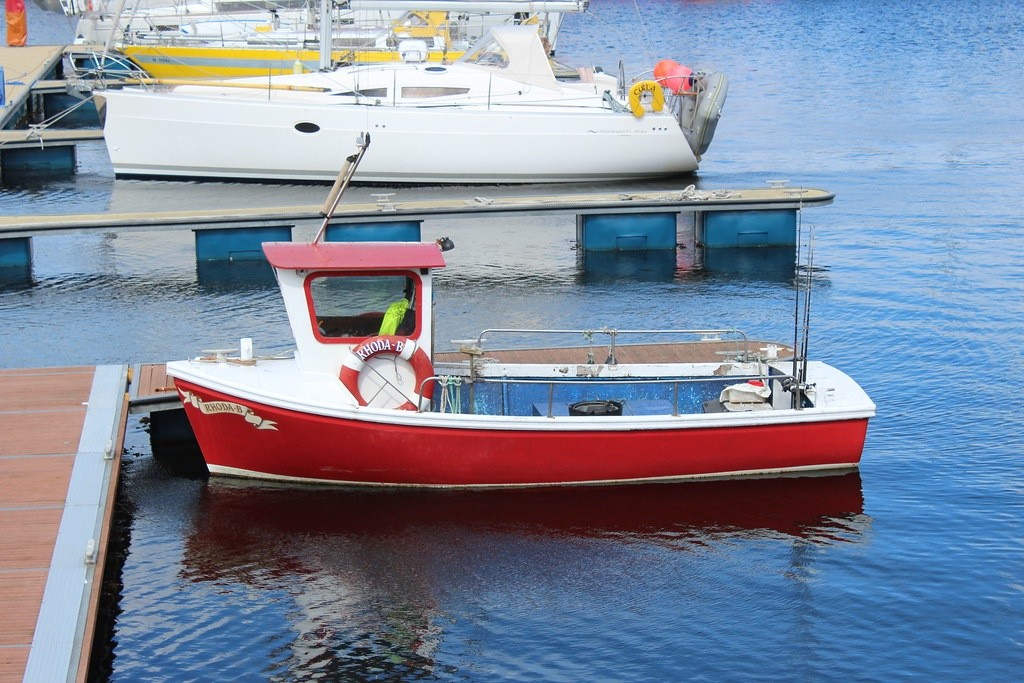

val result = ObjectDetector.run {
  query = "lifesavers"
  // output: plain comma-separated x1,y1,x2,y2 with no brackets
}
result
629,80,665,118
339,334,434,413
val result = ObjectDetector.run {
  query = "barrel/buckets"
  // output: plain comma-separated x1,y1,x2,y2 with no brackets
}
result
566,400,626,416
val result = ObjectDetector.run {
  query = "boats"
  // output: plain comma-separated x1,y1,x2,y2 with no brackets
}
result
58,1,729,186
164,130,877,490
